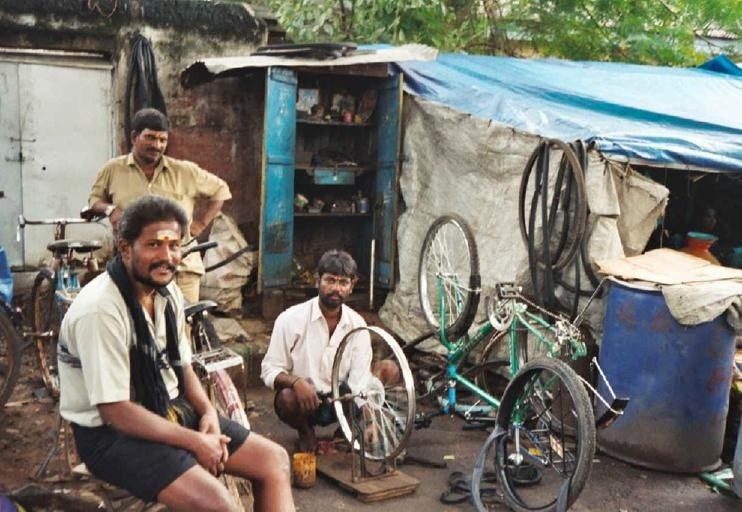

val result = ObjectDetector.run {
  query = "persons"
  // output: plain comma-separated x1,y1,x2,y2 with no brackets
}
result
258,249,402,461
57,195,296,511
663,200,729,268
88,107,233,349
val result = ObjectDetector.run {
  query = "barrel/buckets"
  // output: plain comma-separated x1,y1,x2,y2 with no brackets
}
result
591,274,735,475
730,422,742,497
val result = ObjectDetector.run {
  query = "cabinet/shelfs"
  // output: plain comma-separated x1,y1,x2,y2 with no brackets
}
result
293,71,380,288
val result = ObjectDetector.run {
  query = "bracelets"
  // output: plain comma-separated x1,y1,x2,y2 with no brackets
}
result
105,203,118,218
290,375,302,390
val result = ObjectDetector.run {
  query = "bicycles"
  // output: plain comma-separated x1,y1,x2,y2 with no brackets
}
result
0,294,23,411
172,235,252,432
17,205,120,404
392,210,631,511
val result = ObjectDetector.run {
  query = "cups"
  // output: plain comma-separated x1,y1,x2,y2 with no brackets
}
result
292,452,317,490
